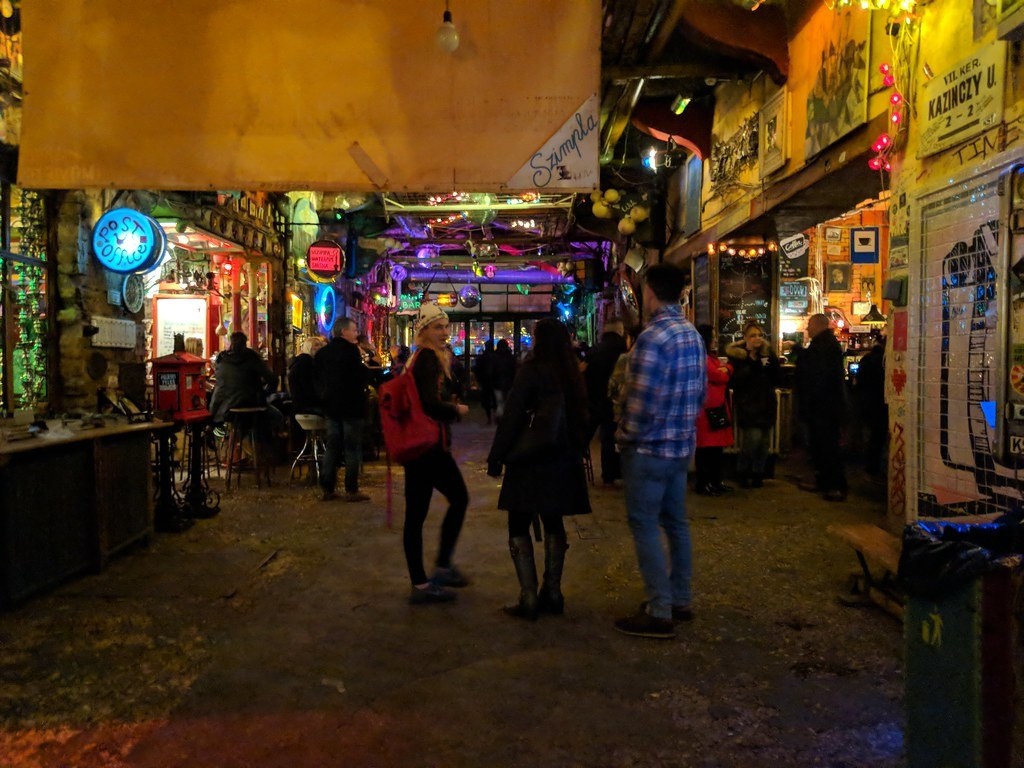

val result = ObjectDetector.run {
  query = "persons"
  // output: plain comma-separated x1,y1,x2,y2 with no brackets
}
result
400,300,470,603
186,313,890,502
487,319,597,619
613,263,708,637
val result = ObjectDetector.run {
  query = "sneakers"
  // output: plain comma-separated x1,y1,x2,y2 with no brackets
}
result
430,564,468,587
612,613,676,639
409,583,457,604
640,602,694,624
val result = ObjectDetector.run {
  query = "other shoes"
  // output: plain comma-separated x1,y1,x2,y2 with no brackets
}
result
344,488,370,501
322,488,340,501
799,481,821,491
825,488,841,502
695,478,725,495
752,475,762,487
737,478,749,489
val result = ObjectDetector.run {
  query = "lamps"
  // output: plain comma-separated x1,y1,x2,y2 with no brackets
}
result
861,304,887,326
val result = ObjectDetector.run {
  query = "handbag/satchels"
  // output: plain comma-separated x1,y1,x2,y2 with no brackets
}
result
501,364,562,464
706,404,730,430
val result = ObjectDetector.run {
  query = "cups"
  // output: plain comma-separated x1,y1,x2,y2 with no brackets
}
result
779,356,785,364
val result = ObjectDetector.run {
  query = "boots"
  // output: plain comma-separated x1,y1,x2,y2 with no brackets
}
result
503,536,539,621
538,534,568,616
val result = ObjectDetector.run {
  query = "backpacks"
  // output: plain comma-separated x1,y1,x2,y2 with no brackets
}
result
377,347,444,463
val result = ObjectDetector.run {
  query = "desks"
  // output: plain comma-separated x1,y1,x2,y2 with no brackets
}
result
0,415,174,618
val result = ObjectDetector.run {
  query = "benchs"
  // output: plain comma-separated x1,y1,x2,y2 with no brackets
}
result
827,521,904,621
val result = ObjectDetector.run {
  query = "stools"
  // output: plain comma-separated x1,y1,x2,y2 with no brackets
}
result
223,407,272,491
180,425,220,481
289,414,327,486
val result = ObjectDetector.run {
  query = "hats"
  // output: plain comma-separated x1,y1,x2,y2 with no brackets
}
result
412,299,448,335
643,264,672,295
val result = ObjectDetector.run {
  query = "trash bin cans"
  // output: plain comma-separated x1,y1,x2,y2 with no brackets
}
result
896,523,1024,768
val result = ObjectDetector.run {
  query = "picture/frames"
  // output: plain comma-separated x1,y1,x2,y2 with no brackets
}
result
685,152,701,235
826,261,853,293
758,83,787,182
850,226,880,264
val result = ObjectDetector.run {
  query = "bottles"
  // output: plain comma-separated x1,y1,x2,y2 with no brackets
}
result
847,333,877,349
